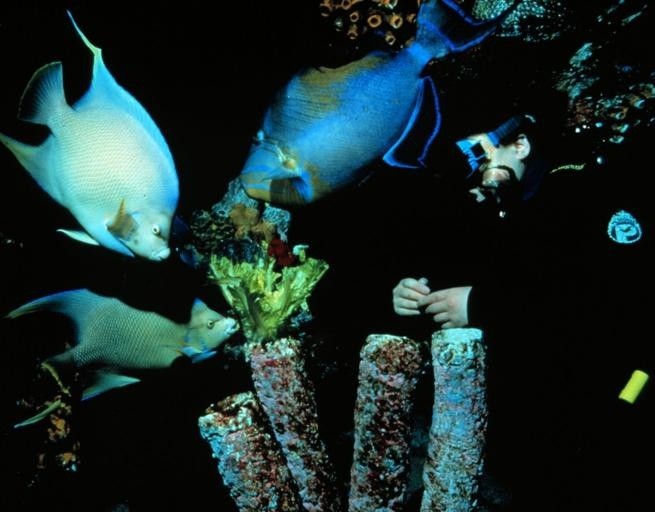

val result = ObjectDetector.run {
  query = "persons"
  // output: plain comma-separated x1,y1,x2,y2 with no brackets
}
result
352,111,645,509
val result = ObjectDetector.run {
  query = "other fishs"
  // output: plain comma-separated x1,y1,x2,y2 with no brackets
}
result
238,1,525,207
1,11,182,264
7,289,241,430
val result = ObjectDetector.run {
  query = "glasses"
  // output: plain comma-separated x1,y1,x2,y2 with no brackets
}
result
451,118,526,179
